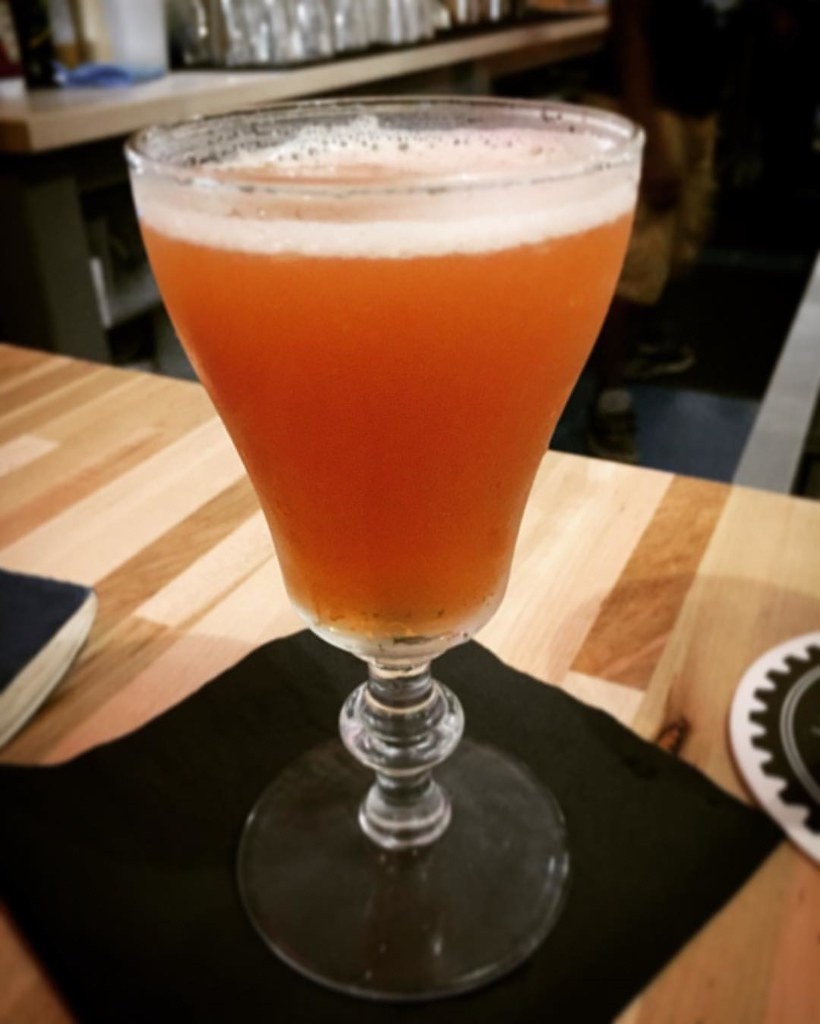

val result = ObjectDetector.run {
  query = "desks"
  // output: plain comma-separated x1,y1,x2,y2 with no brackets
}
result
1,1,611,382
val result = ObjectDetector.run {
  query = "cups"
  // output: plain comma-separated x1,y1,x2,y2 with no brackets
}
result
164,0,506,71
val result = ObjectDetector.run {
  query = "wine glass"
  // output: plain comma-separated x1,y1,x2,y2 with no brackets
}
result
129,95,645,993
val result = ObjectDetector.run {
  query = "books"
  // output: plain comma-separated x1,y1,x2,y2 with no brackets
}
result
0,565,101,750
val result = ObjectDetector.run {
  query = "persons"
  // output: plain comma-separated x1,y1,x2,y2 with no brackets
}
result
581,0,820,462
